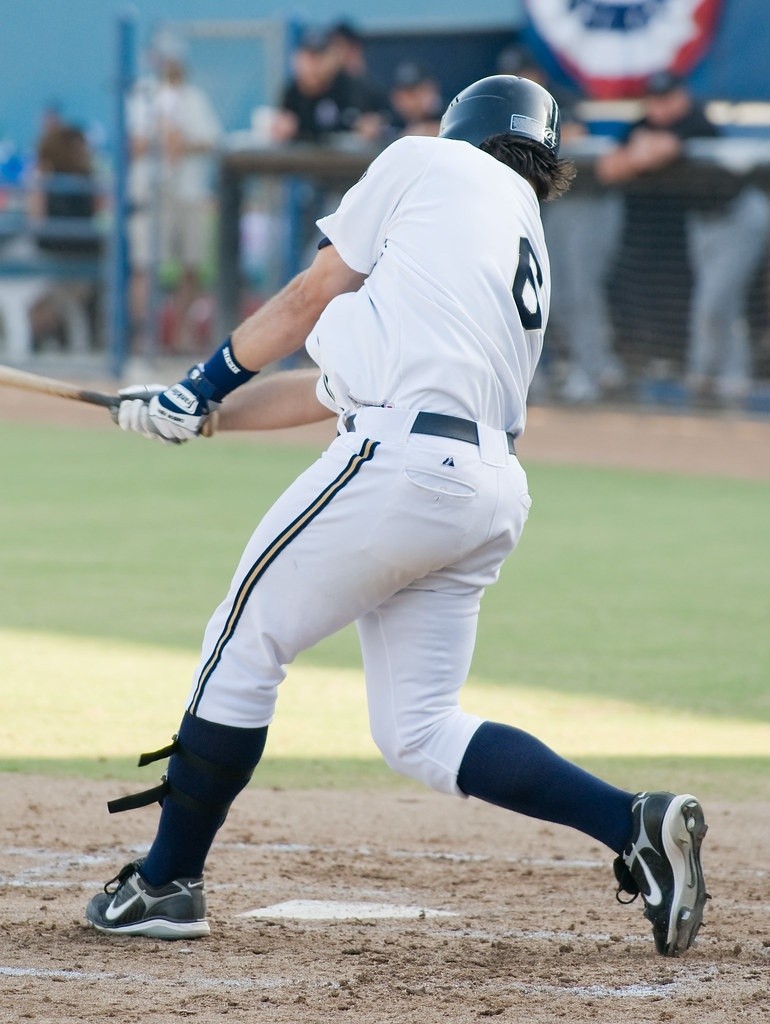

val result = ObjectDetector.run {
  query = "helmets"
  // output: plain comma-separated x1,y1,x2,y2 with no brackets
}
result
440,75,561,150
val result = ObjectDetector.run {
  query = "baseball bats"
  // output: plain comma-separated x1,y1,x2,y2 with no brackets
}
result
0,362,222,437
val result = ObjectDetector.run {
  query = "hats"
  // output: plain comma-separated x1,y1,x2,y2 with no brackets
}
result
650,73,677,94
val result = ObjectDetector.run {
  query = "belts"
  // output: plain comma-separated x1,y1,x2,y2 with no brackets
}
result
338,410,516,454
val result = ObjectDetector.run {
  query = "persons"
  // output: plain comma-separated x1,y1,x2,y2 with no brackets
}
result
87,74,710,959
35,14,768,399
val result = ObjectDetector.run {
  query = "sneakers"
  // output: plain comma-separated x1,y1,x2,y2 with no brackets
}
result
613,790,706,956
86,858,210,938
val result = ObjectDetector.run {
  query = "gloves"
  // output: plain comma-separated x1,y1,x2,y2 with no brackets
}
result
112,382,170,433
147,364,219,443
